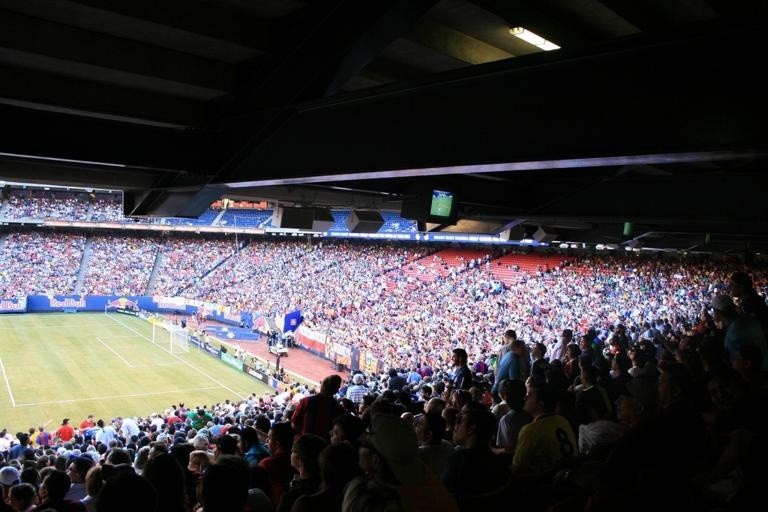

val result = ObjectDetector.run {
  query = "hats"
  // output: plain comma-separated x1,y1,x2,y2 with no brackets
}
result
57,446,96,456
353,374,363,385
0,466,21,487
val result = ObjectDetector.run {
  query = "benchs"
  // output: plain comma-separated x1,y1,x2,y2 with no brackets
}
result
378,248,630,304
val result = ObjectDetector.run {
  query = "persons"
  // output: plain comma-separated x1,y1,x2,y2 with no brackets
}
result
1,401,768,511
0,187,176,298
176,236,768,402
